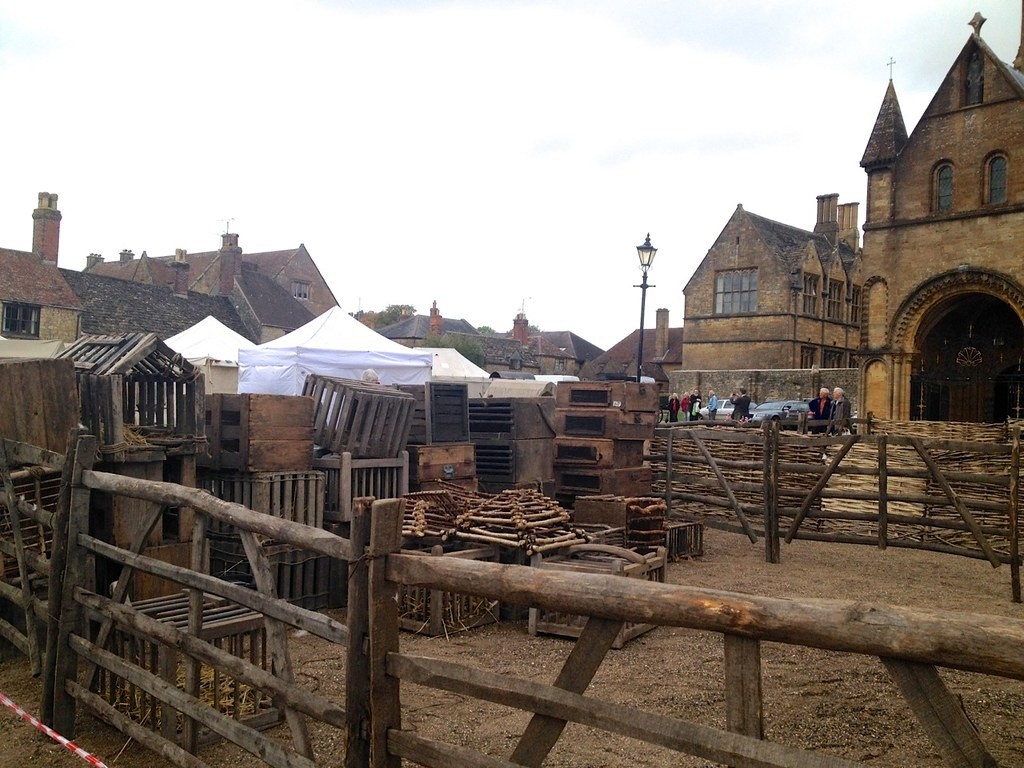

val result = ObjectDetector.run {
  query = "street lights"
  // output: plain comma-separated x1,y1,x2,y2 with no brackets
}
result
632,232,658,383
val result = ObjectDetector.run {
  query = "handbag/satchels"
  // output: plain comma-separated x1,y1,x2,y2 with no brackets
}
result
731,409,742,419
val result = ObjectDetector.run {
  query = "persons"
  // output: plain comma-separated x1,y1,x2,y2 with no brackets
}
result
730,388,749,423
809,386,849,432
663,387,716,421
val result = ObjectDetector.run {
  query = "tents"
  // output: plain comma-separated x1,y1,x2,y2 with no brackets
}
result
124,316,255,426
414,340,491,411
238,305,433,442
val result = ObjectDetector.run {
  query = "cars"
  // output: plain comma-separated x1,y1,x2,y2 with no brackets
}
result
851,411,858,431
698,398,756,420
749,401,814,431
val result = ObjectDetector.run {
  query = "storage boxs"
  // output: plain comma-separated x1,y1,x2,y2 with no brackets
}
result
0,359,705,753
